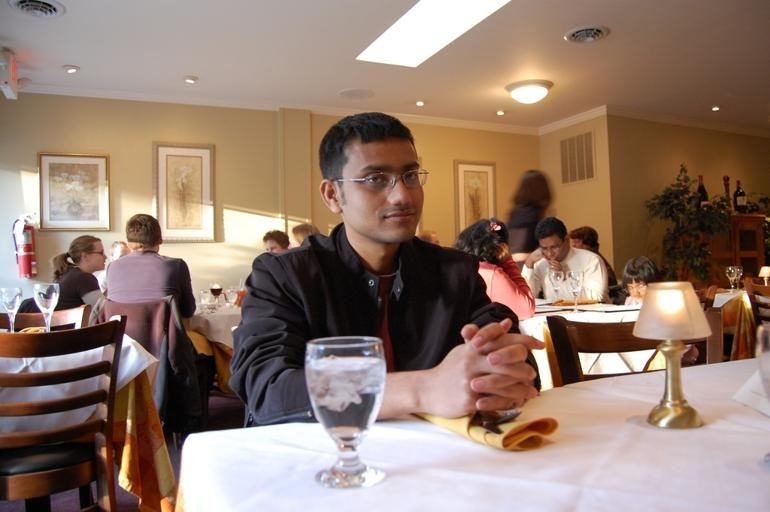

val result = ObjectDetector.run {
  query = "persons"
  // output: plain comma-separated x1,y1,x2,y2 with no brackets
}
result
416,229,439,245
46,235,108,331
568,225,618,298
96,241,131,294
456,217,537,321
263,230,289,254
102,212,196,318
292,223,320,247
521,216,611,303
623,254,699,367
228,111,543,427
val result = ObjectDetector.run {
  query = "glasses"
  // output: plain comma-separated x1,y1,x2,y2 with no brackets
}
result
329,169,428,192
538,239,566,252
87,250,105,256
621,284,645,294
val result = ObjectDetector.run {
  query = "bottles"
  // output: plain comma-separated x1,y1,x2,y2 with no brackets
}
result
696,175,708,211
733,180,748,214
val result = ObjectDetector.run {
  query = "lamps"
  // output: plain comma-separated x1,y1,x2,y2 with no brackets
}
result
504,78,554,105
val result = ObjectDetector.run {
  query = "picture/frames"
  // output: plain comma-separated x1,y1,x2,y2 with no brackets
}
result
37,151,111,233
453,159,497,241
151,141,217,244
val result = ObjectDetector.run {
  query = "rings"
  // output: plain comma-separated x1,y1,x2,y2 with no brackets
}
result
552,265,554,268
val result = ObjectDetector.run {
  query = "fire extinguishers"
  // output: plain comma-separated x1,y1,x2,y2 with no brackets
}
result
12,215,39,279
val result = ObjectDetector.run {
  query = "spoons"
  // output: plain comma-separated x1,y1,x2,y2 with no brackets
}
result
483,410,521,429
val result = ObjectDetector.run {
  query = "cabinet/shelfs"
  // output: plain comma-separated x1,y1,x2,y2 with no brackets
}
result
674,214,768,290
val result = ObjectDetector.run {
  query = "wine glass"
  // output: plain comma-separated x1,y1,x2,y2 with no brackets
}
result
0,287,23,332
303,336,387,489
548,269,585,315
754,324,770,462
198,279,247,314
33,282,61,332
724,265,743,296
